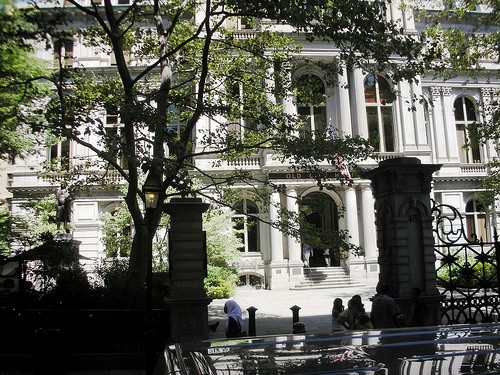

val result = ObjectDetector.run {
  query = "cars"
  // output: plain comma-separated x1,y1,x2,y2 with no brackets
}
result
150,321,500,375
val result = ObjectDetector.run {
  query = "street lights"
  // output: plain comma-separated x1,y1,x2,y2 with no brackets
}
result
142,164,165,375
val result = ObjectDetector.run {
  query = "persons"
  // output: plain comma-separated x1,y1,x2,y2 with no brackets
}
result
302,241,313,267
224,302,243,338
337,295,373,330
332,298,344,331
371,286,400,328
323,249,331,267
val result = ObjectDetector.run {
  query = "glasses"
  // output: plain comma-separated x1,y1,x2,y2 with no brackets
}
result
349,305,355,309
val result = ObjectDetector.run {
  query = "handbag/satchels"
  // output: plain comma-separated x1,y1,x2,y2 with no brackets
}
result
357,313,370,330
236,317,247,337
